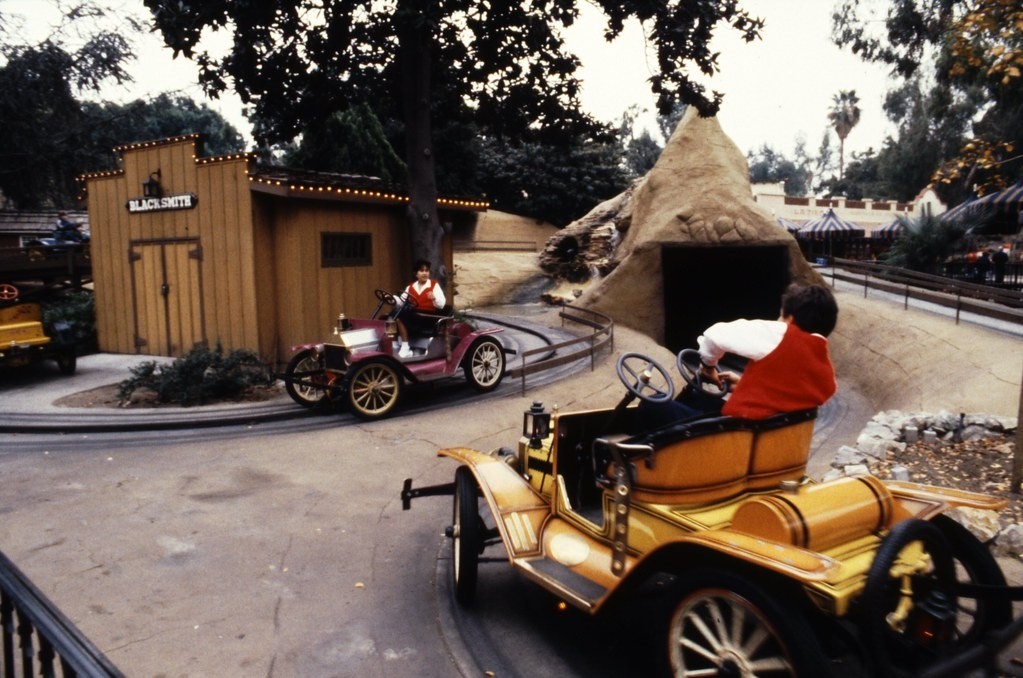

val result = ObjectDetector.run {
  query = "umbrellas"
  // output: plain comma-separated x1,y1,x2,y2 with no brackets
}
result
871,213,916,239
772,208,802,237
939,180,1023,234
796,202,865,287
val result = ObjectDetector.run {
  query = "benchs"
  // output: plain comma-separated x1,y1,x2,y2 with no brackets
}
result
591,407,818,504
414,304,453,335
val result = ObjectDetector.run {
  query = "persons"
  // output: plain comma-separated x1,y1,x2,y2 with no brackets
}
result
699,284,839,422
50,211,86,241
992,247,1009,282
975,252,992,279
381,259,446,358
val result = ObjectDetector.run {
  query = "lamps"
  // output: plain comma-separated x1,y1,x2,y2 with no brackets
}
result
143,169,161,197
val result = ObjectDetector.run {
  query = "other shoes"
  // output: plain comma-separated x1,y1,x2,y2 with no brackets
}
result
398,346,413,357
